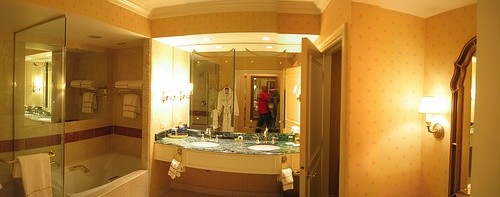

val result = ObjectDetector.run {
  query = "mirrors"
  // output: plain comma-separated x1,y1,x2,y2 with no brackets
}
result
447,35,478,196
250,74,278,120
173,42,300,136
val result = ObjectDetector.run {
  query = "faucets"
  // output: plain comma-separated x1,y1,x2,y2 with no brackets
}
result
205,127,212,140
263,131,268,144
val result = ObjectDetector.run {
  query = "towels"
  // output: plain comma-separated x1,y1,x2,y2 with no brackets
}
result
167,152,183,179
13,153,52,197
277,162,294,191
82,92,97,114
70,80,105,90
122,94,140,118
114,80,143,89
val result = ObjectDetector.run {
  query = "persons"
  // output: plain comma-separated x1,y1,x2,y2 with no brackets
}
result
256,87,275,133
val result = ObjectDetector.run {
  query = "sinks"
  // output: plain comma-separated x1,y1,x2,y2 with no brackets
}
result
190,139,220,147
248,144,281,150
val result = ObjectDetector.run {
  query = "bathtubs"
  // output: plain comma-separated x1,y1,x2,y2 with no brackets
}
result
51,152,149,196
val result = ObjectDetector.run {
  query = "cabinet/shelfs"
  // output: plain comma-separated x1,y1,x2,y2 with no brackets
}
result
191,60,221,129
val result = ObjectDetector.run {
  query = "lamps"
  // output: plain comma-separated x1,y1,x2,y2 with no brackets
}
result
419,97,445,139
